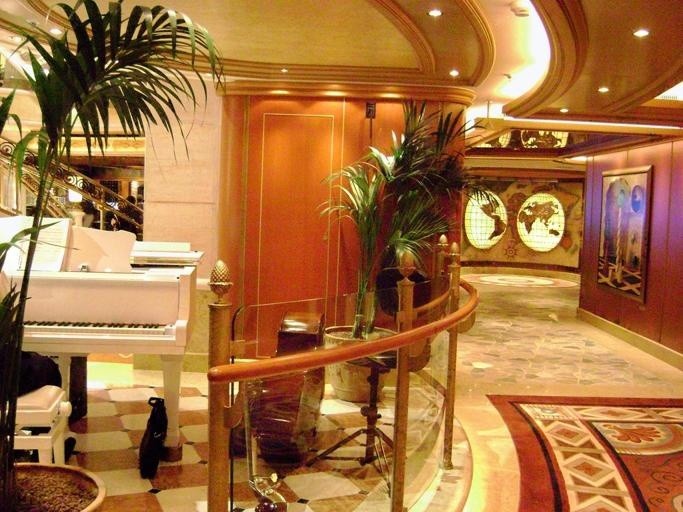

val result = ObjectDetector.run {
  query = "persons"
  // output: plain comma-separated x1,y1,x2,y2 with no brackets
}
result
110,195,143,233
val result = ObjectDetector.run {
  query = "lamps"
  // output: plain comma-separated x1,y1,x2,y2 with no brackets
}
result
0,0,228,512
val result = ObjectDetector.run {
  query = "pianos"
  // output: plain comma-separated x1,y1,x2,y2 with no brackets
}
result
0,215,205,461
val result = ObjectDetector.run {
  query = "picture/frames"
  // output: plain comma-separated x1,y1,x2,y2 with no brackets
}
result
596,165,653,304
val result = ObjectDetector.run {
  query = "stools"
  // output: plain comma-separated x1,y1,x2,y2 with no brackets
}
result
305,350,396,494
5,384,73,465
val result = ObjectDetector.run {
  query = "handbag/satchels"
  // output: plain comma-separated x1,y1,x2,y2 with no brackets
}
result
140,396,167,479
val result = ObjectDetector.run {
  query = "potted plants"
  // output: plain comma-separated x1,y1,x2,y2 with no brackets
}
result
316,95,498,403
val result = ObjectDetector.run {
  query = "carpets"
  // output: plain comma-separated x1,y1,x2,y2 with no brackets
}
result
486,395,683,512
